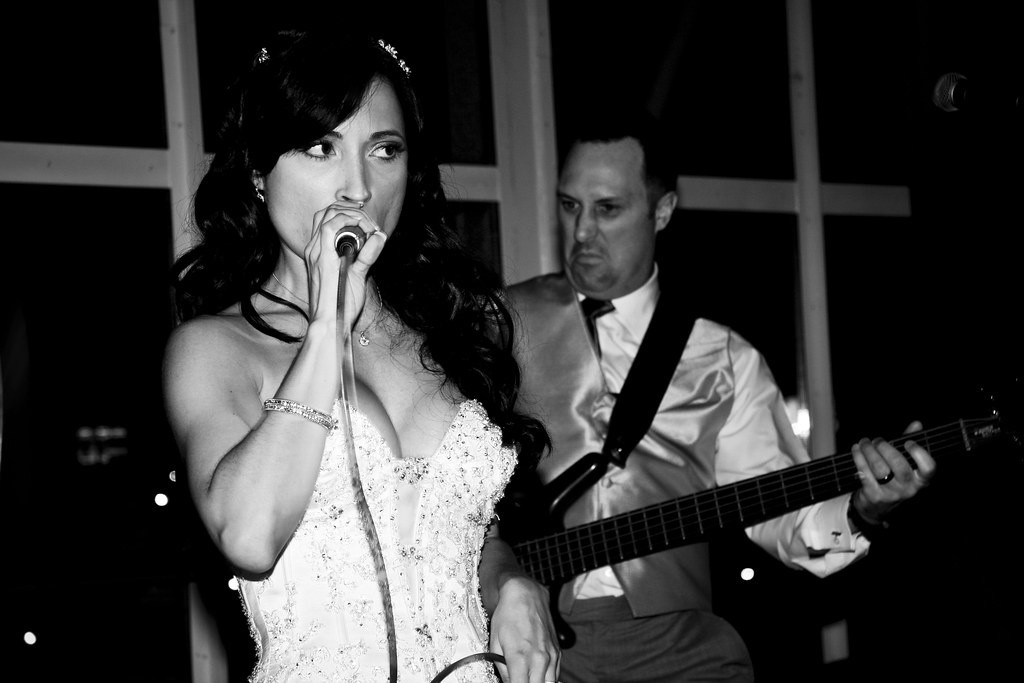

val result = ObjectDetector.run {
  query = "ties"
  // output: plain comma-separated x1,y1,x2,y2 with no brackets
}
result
580,296,615,358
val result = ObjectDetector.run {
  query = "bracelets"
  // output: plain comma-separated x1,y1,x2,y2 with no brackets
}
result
264,398,334,431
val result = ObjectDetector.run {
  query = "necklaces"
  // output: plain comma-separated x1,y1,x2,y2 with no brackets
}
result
269,271,383,345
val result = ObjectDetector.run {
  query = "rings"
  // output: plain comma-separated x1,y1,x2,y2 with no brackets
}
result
876,470,894,484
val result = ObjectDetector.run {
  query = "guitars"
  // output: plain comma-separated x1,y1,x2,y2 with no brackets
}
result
496,372,1024,651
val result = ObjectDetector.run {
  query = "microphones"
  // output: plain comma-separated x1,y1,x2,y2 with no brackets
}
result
334,225,367,257
934,72,1024,111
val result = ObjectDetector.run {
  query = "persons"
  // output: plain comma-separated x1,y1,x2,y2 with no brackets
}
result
161,24,562,683
468,98,937,683
